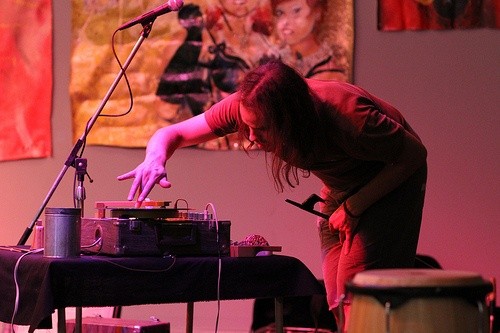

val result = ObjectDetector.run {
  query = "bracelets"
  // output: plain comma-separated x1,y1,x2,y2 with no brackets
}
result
343,197,363,219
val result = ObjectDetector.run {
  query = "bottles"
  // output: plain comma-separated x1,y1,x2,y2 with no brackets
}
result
33,219,44,249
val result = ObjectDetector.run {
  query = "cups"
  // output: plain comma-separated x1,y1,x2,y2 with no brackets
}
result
44,206,82,259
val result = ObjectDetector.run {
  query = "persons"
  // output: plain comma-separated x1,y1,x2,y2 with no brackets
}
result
117,62,428,333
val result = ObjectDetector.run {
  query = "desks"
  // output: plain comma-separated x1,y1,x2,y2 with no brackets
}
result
0,246,330,332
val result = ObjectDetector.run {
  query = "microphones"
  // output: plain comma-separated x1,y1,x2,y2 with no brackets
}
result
117,0,183,30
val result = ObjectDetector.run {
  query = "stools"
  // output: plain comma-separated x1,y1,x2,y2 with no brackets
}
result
61,317,170,332
